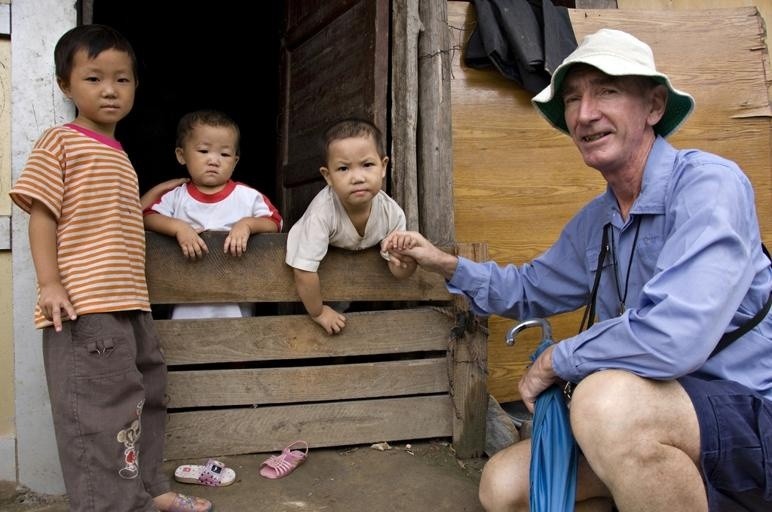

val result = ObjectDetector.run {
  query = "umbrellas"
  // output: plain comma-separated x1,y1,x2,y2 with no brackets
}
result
502,315,580,512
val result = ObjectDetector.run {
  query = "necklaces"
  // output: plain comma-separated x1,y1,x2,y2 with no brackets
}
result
608,212,642,316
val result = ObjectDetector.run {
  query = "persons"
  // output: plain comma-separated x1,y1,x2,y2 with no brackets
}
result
137,105,283,409
381,27,772,511
282,119,419,367
7,21,214,510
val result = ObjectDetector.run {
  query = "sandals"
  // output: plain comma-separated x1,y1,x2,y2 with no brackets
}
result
259,439,310,480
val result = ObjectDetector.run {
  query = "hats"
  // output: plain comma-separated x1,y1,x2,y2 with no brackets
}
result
532,29,695,143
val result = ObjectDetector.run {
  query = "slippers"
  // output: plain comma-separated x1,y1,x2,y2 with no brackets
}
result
163,492,213,512
174,458,236,486
